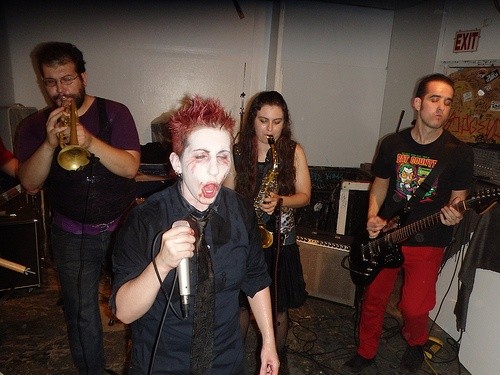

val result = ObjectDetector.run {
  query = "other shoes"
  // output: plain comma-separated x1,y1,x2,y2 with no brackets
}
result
278,344,290,375
399,344,424,374
342,352,375,374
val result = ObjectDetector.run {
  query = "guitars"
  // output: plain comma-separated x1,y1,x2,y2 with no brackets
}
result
349,186,500,287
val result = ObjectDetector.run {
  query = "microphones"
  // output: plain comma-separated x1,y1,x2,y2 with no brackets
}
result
171,220,192,318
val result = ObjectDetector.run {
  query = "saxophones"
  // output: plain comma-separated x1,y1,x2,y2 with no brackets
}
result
253,135,280,249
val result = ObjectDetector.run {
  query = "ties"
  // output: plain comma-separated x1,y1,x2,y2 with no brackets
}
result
174,182,222,375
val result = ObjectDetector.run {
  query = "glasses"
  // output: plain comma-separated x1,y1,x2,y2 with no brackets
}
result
43,72,80,86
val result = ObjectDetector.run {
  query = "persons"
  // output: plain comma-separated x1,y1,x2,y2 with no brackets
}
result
341,74,474,375
13,43,140,375
107,94,280,375
0,135,17,179
222,90,311,375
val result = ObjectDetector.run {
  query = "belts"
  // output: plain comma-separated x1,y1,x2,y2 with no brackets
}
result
48,200,138,236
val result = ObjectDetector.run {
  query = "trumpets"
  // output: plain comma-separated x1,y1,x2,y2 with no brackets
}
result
57,95,92,172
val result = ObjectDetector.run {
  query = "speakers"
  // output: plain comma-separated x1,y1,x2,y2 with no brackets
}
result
0,214,42,293
295,238,361,307
